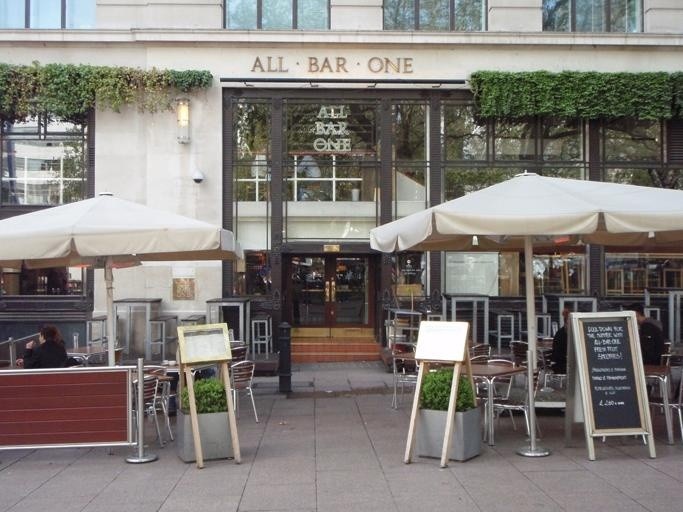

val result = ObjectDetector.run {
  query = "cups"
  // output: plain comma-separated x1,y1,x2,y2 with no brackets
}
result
551,322,558,337
72,333,78,343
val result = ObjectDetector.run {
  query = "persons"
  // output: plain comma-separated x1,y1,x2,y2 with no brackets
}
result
15,325,68,368
624,303,664,365
297,149,322,201
250,146,272,201
551,308,571,374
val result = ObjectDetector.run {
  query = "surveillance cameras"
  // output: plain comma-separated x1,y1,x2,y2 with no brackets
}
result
192,171,204,183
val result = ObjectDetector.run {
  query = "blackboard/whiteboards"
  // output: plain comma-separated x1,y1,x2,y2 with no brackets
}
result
572,311,652,436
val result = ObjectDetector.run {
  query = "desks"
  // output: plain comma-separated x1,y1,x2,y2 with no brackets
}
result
63,342,125,366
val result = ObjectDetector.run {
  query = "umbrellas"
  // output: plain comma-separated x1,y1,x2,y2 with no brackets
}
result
369,168,682,456
0,192,241,367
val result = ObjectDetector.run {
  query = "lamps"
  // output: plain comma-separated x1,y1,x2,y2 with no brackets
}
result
172,95,192,147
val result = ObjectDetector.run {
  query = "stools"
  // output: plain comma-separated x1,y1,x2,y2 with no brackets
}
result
382,293,662,352
82,294,274,365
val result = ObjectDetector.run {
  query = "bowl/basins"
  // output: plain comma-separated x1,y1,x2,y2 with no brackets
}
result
168,361,175,365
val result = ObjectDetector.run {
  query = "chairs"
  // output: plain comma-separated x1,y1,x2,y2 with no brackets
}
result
119,341,261,450
385,329,683,453
3,141,68,205
